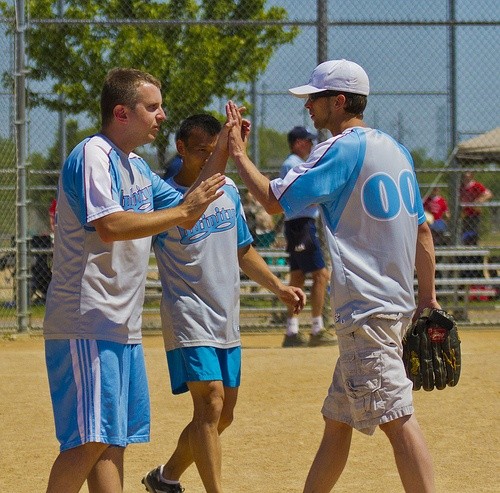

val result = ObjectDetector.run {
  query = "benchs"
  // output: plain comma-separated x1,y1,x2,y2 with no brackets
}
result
66,242,498,334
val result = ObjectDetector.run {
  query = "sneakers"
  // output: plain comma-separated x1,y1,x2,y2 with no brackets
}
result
282,333,302,344
142,467,184,493
311,330,337,346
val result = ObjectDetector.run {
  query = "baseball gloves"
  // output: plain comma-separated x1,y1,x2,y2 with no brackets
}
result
402,307,462,392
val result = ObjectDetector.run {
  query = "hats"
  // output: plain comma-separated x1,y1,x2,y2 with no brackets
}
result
290,57,369,96
288,124,318,140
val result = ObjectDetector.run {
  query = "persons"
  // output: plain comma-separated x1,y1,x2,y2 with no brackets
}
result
43,67,252,493
140,115,307,492
50,170,492,303
226,60,444,492
281,127,340,347
